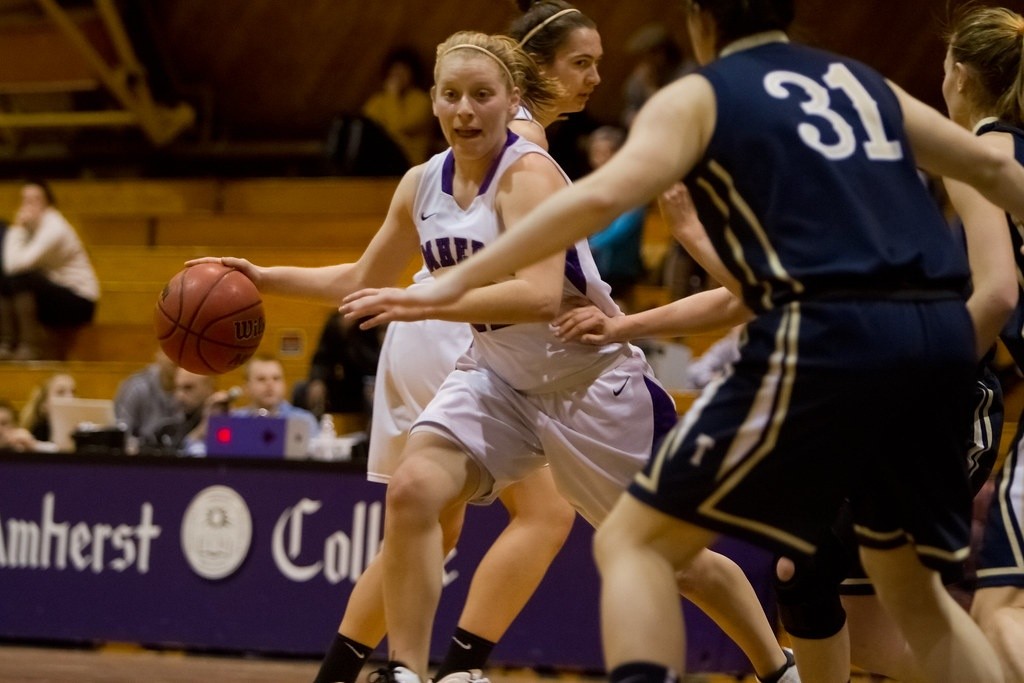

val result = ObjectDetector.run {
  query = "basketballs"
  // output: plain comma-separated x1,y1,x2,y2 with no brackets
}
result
151,262,267,377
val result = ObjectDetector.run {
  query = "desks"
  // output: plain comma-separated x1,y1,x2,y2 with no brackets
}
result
1,451,779,682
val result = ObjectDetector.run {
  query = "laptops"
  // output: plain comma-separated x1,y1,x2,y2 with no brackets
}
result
205,410,286,458
50,396,117,453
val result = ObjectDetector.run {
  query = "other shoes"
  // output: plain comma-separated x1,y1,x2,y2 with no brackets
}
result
753,647,802,683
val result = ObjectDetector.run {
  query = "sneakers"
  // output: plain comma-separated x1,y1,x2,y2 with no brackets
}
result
366,661,423,683
426,668,491,683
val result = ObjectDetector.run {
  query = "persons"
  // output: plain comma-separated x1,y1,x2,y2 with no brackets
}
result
0,350,320,458
0,182,101,360
337,45,434,179
183,0,1024,683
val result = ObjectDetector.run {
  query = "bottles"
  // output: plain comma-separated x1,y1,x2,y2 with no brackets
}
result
321,415,334,461
